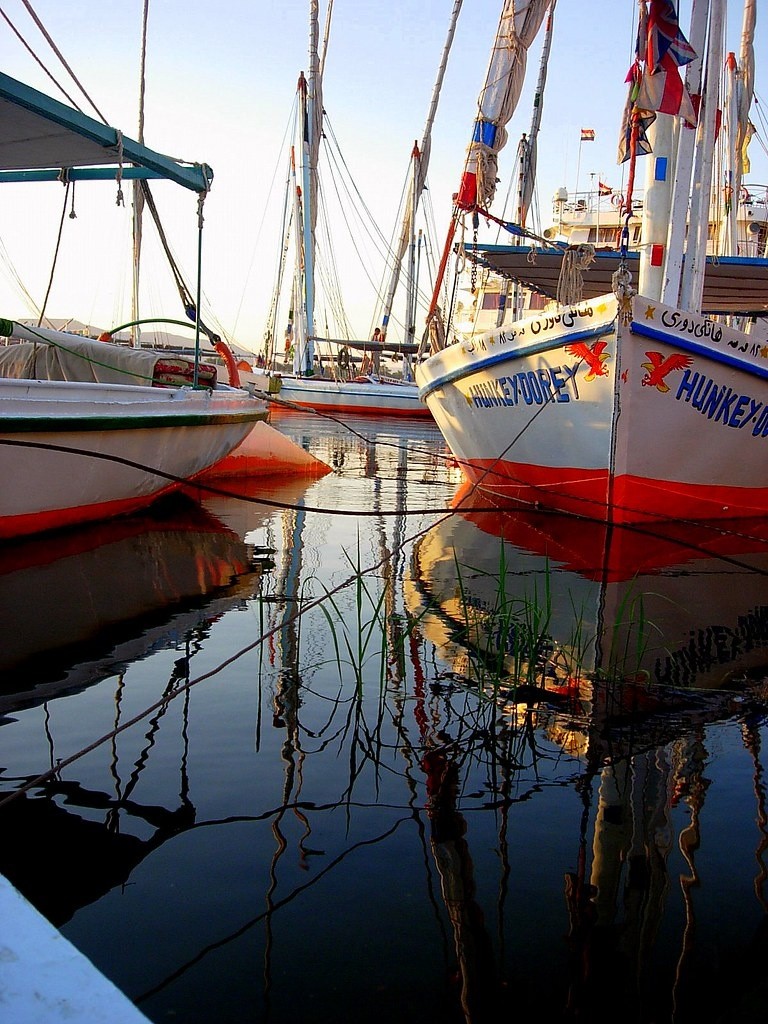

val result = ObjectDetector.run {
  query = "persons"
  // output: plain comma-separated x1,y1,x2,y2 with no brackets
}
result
370,328,383,375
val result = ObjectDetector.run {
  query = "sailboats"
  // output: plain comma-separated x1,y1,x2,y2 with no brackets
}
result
0,0,768,532
402,483,768,786
0,493,276,726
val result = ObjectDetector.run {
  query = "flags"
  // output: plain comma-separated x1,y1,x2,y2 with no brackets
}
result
616,0,697,165
598,182,612,197
581,129,595,141
